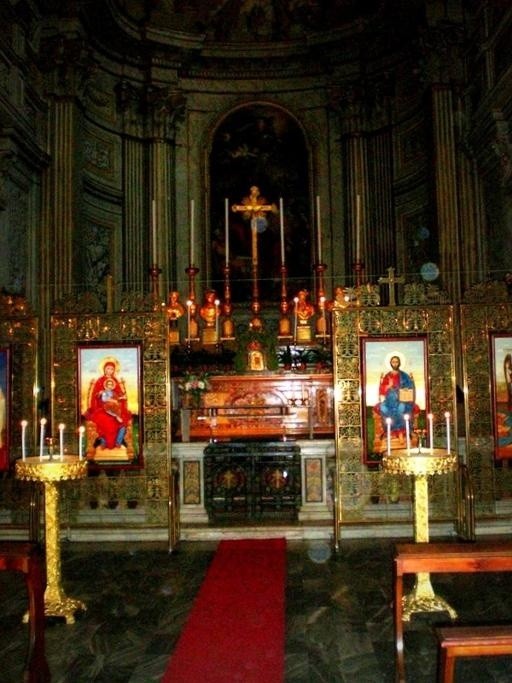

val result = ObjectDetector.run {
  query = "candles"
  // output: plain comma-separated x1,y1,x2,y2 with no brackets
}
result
150,198,158,271
21,423,86,464
278,195,287,268
189,198,196,268
293,310,328,349
354,194,362,267
184,306,225,347
386,416,453,459
224,198,230,269
315,194,323,265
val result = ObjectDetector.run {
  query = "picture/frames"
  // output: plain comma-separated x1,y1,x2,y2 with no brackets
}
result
359,332,434,466
74,338,145,472
487,329,512,462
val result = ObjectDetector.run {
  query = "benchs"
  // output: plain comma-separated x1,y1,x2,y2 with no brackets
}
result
435,626,512,681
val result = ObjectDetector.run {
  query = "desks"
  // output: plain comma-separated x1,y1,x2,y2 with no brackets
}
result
389,538,510,683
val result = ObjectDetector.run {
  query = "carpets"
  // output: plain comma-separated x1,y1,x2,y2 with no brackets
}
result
161,536,289,682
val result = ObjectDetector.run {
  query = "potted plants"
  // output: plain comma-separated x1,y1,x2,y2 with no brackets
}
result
279,346,328,370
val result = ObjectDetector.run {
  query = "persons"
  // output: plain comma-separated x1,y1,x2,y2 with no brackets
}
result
90,355,127,450
100,377,122,422
378,354,415,439
163,285,346,318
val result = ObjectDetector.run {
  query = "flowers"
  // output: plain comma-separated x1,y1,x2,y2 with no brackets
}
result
177,370,210,394
